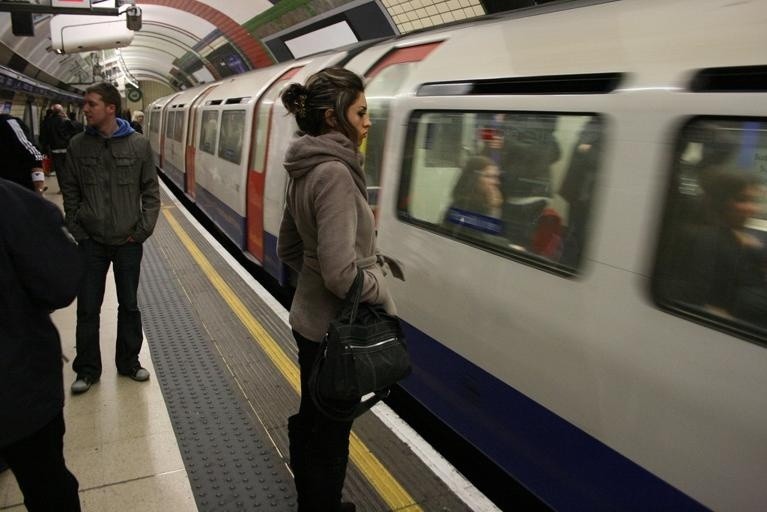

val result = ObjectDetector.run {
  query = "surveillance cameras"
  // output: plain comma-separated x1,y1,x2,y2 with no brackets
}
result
125,5,143,30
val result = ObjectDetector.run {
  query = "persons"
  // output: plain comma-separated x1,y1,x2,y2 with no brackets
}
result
129,110,144,134
0,178,82,512
275,67,398,512
60,82,160,394
0,114,45,196
39,104,84,191
688,173,767,331
440,112,600,273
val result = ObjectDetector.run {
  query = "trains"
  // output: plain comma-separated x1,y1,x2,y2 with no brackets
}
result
134,0,767,512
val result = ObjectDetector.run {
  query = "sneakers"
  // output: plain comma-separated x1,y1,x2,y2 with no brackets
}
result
129,366,148,381
71,375,92,393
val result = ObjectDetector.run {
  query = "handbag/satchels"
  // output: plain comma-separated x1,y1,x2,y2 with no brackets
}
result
321,312,411,400
